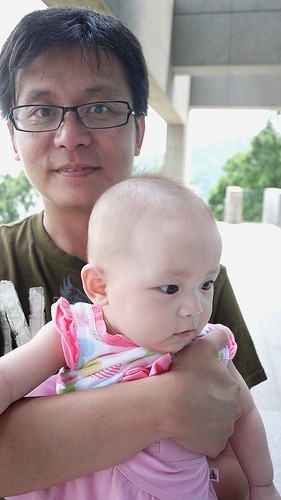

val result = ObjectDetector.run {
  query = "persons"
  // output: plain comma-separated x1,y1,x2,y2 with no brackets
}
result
0,8,267,500
1,170,280,500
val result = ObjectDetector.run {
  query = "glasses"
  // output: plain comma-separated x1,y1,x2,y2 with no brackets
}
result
8,99,148,132
